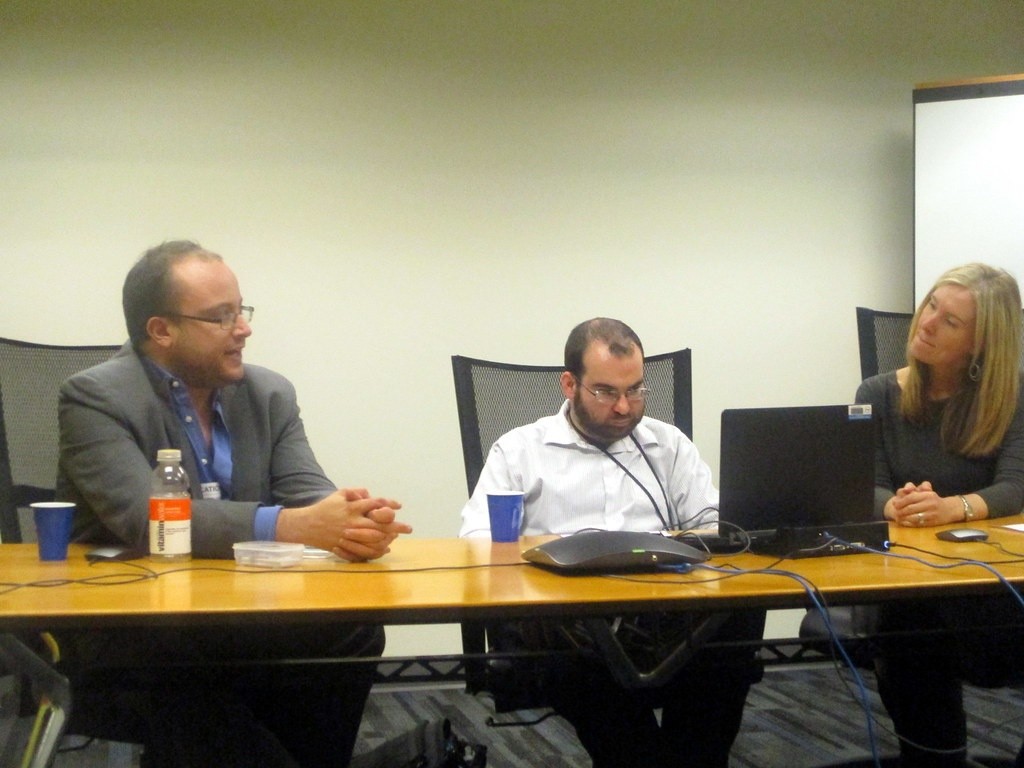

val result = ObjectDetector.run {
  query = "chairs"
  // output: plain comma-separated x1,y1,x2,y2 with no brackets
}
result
856,306,913,381
452,348,765,712
0,336,145,753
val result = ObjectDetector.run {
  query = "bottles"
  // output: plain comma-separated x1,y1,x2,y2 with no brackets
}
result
150,449,193,564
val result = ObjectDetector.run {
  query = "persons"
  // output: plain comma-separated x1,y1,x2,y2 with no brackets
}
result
853,263,1024,768
57,240,412,768
460,316,749,768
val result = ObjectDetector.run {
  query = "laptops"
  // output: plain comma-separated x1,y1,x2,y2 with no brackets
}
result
667,401,874,544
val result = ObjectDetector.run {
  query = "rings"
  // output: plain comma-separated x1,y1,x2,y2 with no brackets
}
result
918,513,923,523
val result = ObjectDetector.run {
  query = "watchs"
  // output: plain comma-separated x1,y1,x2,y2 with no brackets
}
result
959,494,975,522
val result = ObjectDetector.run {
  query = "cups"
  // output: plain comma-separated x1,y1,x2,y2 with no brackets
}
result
488,490,525,543
29,502,76,562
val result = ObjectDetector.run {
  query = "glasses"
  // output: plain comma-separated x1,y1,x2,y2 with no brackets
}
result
158,305,255,330
570,371,652,404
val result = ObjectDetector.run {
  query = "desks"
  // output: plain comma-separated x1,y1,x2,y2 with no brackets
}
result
0,513,1024,768
